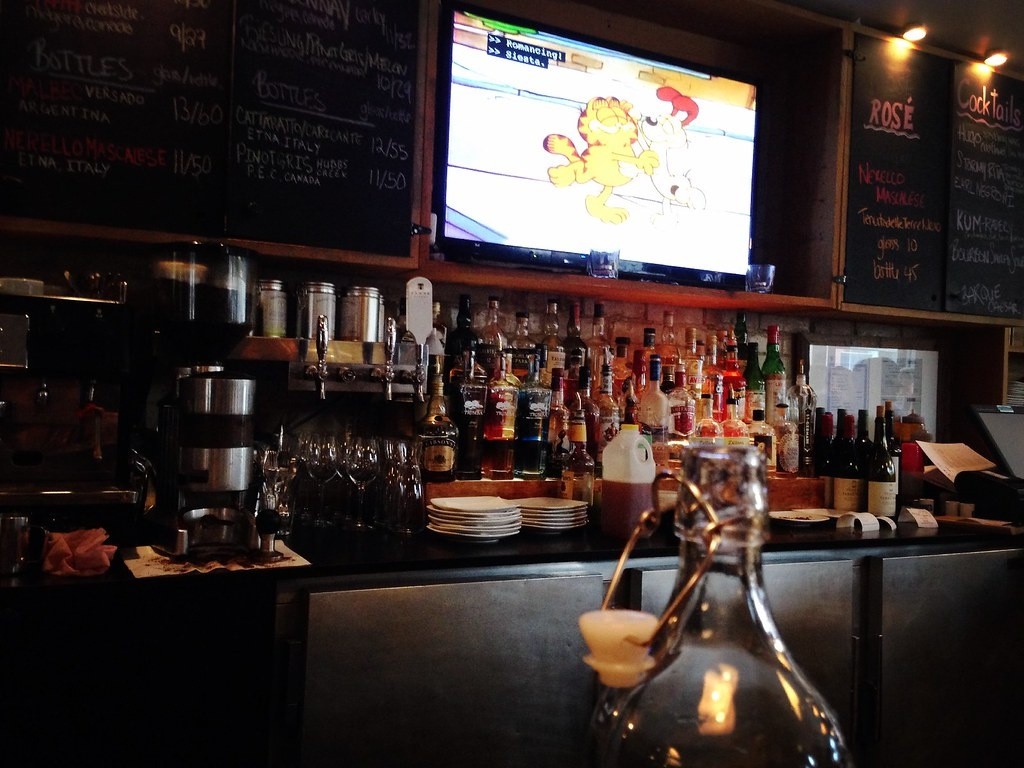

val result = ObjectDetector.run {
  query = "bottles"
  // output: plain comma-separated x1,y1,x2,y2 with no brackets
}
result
381,439,423,534
423,296,930,539
584,440,847,768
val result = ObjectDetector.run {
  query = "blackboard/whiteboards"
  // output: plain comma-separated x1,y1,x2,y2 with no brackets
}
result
839,30,1024,322
1,0,429,269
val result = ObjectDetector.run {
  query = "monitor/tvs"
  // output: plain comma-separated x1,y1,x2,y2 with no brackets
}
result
433,0,761,293
971,404,1024,483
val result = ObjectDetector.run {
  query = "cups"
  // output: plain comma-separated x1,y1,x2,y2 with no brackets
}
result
0,512,49,587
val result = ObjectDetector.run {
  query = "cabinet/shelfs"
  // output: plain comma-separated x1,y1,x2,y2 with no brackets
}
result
305,547,1024,768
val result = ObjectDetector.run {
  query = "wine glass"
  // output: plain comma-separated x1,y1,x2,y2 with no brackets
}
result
253,432,380,537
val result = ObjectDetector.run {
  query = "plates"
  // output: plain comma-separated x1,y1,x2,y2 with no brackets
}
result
769,508,831,524
427,495,590,543
